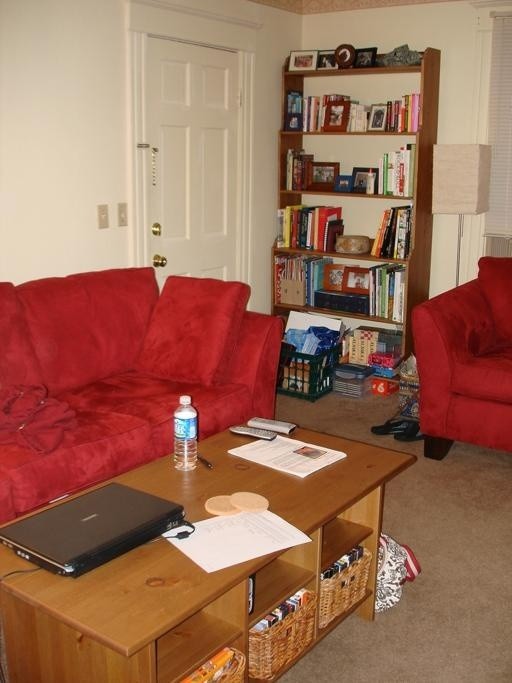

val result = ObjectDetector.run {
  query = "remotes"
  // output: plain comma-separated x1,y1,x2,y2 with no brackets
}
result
229,425,278,441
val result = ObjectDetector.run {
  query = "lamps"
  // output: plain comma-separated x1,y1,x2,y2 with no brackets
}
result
432,144,491,286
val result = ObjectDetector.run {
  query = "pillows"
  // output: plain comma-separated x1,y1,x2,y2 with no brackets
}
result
478,256,512,347
140,276,251,388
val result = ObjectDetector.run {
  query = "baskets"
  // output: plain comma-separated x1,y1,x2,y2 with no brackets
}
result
316,548,372,630
213,646,247,683
346,331,400,367
276,343,338,403
247,591,317,679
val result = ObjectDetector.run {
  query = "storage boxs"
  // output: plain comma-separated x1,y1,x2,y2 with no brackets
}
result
372,377,400,397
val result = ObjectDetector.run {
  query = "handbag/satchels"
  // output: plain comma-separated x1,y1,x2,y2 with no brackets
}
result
374,532,422,614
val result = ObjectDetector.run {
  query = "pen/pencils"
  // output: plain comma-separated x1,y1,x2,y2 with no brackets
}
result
197,455,212,469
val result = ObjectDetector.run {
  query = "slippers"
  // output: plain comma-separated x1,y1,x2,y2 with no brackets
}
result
371,418,408,435
394,422,422,441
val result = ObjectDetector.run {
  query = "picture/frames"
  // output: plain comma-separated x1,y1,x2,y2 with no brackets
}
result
352,168,378,194
367,104,387,131
288,50,319,71
324,101,350,132
335,176,353,192
335,44,356,68
353,48,377,68
324,265,343,291
317,50,339,70
306,162,339,191
342,267,369,295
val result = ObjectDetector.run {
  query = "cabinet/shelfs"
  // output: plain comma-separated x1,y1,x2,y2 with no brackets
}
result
273,47,441,378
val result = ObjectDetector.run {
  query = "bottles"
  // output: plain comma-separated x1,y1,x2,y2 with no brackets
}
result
174,395,197,472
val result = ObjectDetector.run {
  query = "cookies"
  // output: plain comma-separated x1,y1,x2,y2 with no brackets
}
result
230,492,269,514
204,495,239,517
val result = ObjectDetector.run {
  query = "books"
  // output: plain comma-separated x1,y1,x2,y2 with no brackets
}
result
286,90,420,132
180,646,238,683
370,205,410,260
379,144,416,197
320,545,364,588
277,205,342,251
285,148,314,191
274,254,333,307
368,263,406,323
255,588,309,630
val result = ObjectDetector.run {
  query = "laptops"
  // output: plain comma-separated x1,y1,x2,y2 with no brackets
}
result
0,482,185,579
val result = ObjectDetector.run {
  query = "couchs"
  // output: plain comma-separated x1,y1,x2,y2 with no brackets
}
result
0,268,285,524
412,259,512,459
